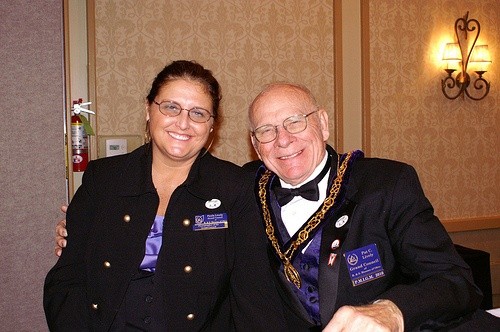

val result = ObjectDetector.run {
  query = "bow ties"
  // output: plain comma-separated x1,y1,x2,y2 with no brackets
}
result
273,153,331,207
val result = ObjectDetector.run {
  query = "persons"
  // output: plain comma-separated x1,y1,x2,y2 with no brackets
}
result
42,59,288,332
54,81,485,332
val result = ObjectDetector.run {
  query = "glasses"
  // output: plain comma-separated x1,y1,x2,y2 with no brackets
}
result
252,108,320,144
152,99,216,123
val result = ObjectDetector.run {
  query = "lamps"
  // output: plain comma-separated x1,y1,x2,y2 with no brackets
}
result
441,11,491,100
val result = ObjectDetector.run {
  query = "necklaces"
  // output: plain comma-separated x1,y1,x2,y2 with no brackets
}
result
259,152,352,289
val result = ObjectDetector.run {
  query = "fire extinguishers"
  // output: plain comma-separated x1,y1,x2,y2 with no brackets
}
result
71,98,95,173
